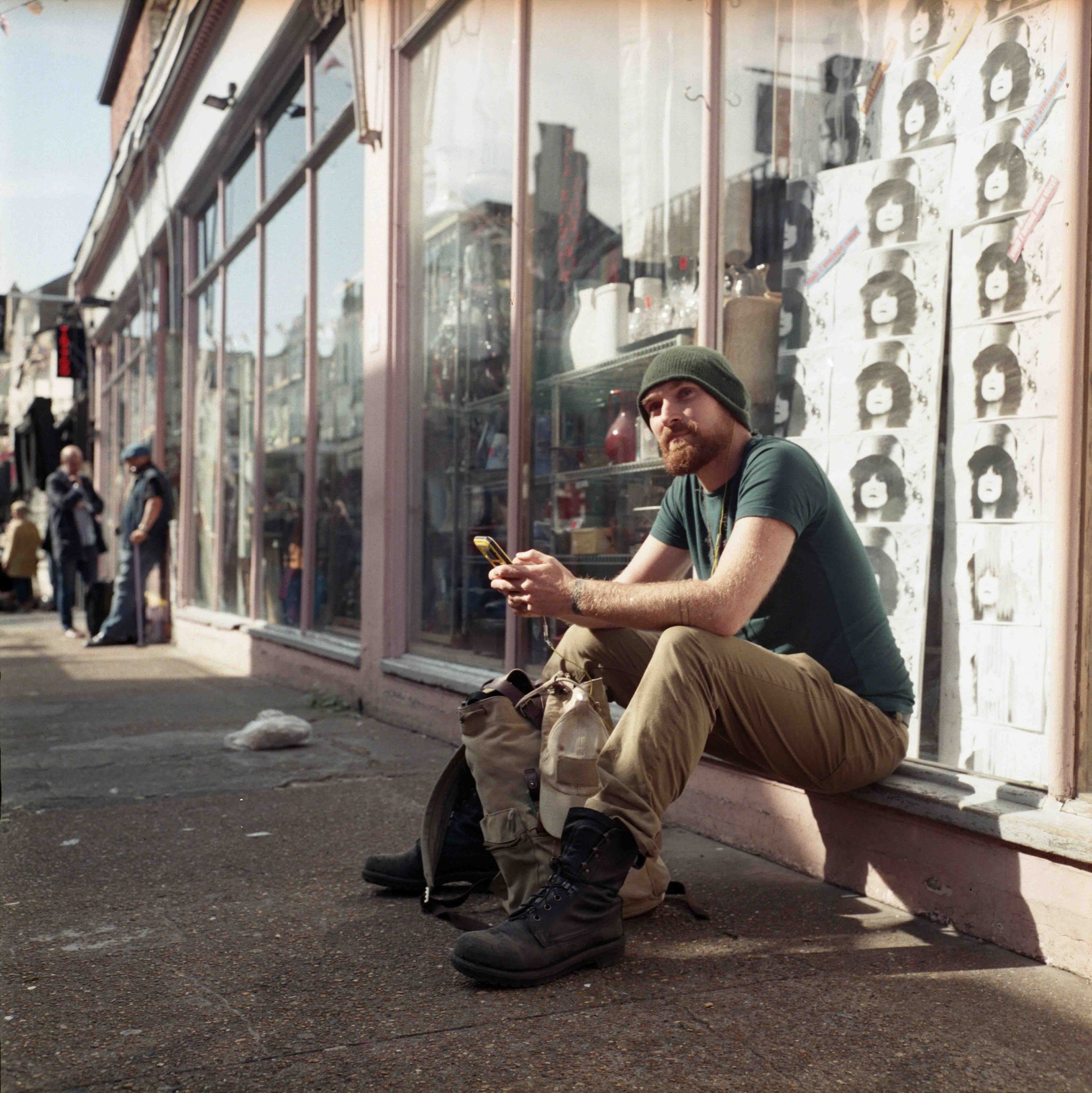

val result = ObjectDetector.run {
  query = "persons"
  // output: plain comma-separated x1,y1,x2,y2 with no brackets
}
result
0,498,43,610
83,443,177,647
45,443,107,640
363,343,914,988
770,0,1036,618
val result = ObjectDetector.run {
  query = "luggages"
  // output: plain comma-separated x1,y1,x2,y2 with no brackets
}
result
84,580,146,645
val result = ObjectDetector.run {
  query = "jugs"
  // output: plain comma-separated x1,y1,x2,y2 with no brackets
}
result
724,264,772,297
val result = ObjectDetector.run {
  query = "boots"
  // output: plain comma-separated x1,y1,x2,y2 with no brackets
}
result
449,806,647,989
361,776,499,893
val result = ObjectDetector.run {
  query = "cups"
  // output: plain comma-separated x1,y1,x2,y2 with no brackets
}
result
636,416,659,463
595,283,631,364
631,277,663,343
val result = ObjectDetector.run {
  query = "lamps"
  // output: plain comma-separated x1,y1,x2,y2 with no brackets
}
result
203,83,236,110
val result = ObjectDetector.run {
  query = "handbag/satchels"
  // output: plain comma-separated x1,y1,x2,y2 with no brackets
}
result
143,591,163,644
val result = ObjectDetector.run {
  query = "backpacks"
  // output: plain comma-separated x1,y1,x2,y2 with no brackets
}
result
418,669,711,933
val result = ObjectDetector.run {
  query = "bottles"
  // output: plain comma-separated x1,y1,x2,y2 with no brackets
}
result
569,288,598,370
605,389,638,464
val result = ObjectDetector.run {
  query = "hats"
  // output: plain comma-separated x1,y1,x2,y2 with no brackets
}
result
120,443,148,458
636,345,752,432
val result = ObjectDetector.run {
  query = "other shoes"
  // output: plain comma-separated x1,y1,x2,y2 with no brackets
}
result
82,631,113,646
65,627,80,639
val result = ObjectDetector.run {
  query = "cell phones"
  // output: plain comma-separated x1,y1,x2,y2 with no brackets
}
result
472,536,512,568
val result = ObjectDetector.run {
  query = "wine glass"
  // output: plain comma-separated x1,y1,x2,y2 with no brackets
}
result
664,255,697,330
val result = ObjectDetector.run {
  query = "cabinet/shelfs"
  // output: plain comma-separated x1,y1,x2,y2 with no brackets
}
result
460,332,693,642
